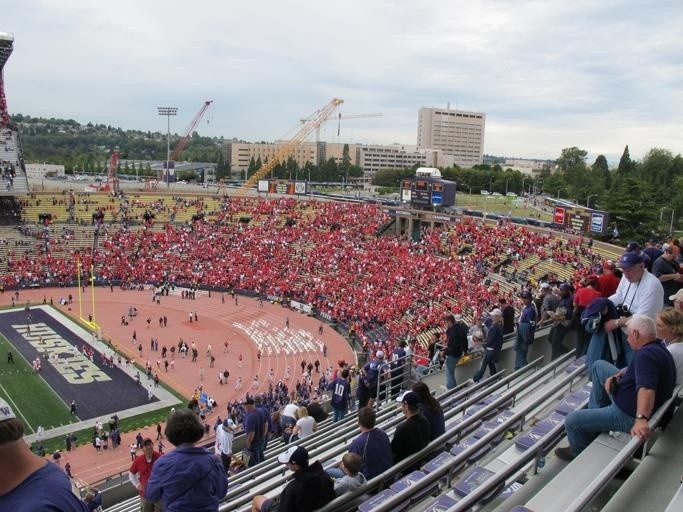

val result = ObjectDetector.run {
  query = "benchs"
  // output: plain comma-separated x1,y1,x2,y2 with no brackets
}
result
104,345,683,512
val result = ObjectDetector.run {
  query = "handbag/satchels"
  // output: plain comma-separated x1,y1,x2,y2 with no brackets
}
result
364,369,377,389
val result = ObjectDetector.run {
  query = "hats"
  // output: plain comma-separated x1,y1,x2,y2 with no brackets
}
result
142,438,152,445
618,252,643,268
376,351,384,358
396,391,422,405
241,398,255,404
278,446,309,463
223,418,237,431
669,288,683,301
488,309,503,316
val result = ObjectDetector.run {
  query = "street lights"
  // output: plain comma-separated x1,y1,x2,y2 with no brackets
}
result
587,194,598,207
339,174,344,185
663,207,674,237
271,167,312,181
557,188,568,198
461,176,545,208
157,106,178,192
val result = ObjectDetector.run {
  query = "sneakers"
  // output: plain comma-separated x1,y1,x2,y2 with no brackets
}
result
555,447,574,461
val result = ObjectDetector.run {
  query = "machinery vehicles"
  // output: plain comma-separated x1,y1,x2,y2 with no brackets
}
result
85,151,120,193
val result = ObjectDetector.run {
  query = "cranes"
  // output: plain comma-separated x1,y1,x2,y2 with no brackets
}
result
299,109,384,142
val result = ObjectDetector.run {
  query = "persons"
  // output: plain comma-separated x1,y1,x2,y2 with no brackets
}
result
0,124,683,511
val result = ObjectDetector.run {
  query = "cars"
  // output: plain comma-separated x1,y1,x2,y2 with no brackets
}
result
49,171,258,188
479,185,557,214
